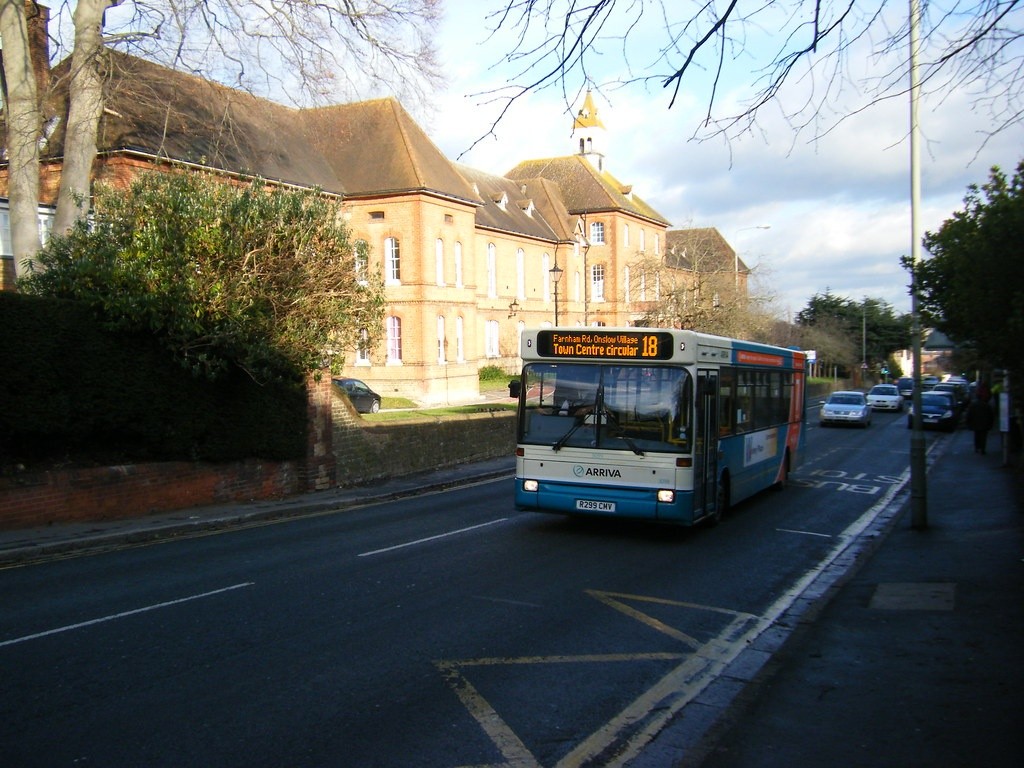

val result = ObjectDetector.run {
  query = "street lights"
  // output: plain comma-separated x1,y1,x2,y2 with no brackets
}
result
860,303,881,388
549,261,565,328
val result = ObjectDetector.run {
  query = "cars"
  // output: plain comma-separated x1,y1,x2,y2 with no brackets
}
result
907,390,964,432
895,377,914,398
818,390,873,428
921,375,939,389
865,383,905,412
933,375,977,410
332,375,382,415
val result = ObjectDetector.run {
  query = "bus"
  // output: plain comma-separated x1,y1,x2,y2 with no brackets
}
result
507,324,810,532
